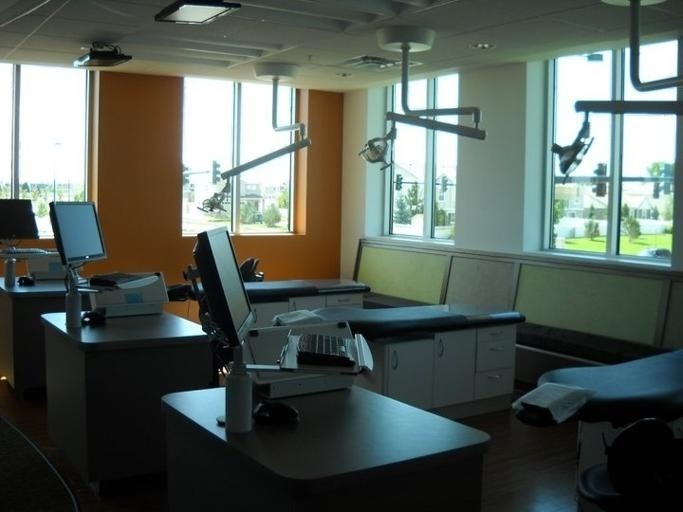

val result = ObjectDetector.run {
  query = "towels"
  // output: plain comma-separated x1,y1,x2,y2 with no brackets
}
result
511,382,597,425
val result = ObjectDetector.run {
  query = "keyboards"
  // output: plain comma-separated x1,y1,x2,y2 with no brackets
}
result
3,247,46,253
89,272,141,287
296,333,356,369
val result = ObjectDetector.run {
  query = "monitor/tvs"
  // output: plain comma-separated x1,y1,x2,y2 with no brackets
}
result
48,200,107,265
0,198,40,240
193,226,255,348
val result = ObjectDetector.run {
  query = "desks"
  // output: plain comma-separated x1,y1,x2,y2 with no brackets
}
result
39,305,216,479
164,376,491,507
0,277,97,388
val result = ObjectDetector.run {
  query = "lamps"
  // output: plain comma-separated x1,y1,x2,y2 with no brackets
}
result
356,28,491,169
158,5,240,26
548,2,682,180
462,25,497,52
197,64,320,218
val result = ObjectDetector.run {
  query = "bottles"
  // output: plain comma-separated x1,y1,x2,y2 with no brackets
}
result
4,252,16,288
225,362,254,433
64,280,83,329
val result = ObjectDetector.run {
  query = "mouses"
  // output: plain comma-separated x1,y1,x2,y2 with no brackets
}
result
81,312,106,325
253,401,300,419
20,276,35,285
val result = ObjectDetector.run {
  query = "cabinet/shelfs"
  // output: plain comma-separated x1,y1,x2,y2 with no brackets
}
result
386,327,476,411
249,293,365,335
475,325,516,399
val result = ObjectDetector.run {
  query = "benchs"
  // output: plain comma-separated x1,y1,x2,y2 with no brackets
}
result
538,346,683,512
